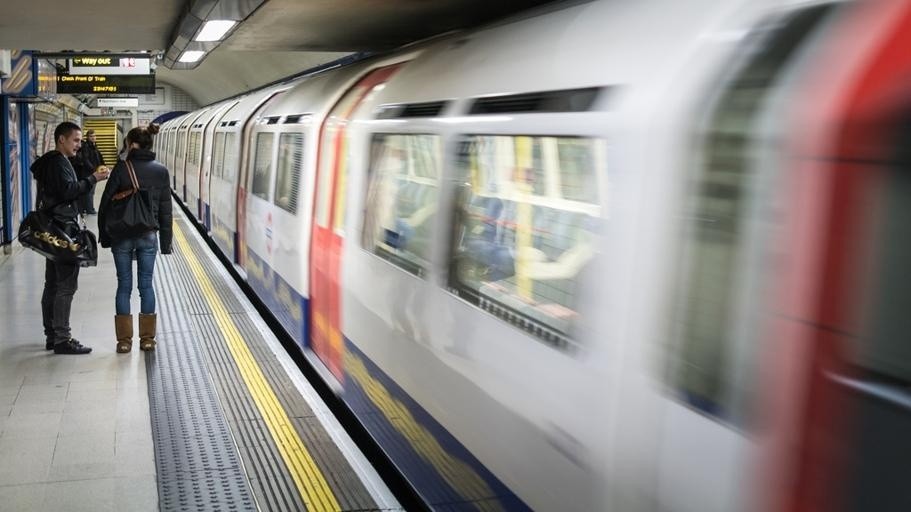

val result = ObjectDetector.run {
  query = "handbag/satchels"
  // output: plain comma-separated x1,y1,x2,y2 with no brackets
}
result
18,211,98,267
98,185,160,247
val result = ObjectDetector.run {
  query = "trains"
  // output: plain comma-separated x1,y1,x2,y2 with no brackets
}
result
146,0,911,512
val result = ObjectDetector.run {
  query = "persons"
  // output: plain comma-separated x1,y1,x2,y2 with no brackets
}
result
97,122,175,354
29,120,111,356
81,129,106,215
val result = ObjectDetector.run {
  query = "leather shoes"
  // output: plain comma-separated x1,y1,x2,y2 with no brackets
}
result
46,337,92,354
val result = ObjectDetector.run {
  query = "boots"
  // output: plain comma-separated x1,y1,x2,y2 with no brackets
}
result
115,315,133,353
139,312,157,351
